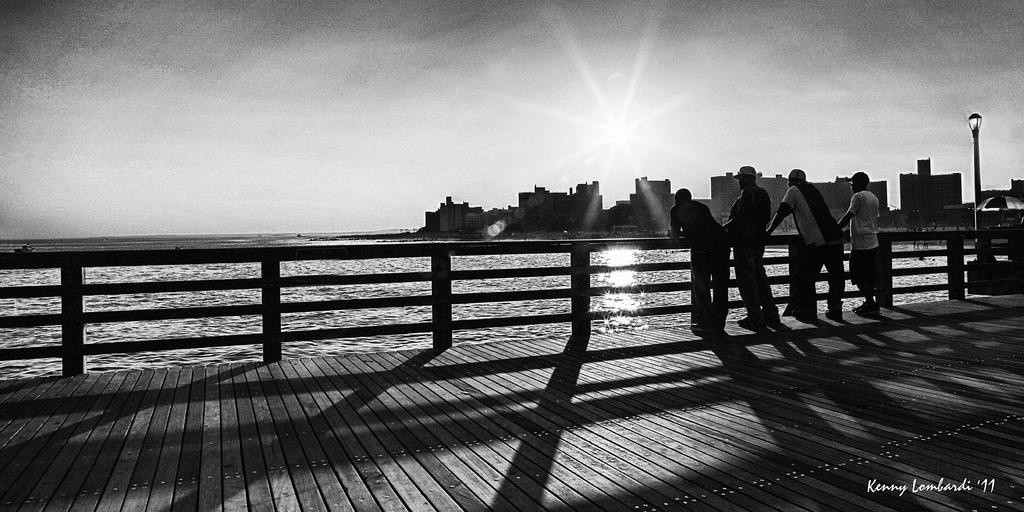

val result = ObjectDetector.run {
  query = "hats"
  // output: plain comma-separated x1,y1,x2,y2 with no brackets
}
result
845,172,869,185
732,166,762,181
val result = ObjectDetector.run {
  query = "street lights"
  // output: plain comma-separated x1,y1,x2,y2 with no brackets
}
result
968,112,984,261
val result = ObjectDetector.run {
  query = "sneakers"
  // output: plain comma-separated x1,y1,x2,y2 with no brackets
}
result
689,296,881,335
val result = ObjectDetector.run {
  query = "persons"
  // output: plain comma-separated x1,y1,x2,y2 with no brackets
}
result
837,172,883,316
721,167,782,325
766,169,848,322
669,188,730,333
